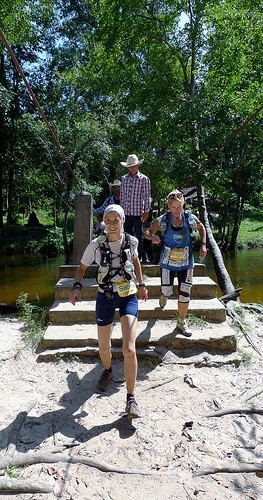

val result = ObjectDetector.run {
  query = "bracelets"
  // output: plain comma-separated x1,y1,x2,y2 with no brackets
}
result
150,233,155,239
73,282,82,289
200,241,207,246
137,284,145,288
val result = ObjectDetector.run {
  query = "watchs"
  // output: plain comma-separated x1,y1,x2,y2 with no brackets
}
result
73,285,82,292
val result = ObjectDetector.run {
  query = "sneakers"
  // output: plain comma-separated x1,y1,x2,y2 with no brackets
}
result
125,397,142,418
159,296,167,308
177,321,192,336
97,370,112,391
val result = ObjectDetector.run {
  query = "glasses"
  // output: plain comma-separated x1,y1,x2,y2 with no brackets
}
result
169,193,182,199
112,186,120,189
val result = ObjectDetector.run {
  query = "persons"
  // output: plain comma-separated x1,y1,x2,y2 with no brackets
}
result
143,189,208,336
95,212,106,237
92,179,122,216
142,194,154,263
68,202,149,419
120,153,151,264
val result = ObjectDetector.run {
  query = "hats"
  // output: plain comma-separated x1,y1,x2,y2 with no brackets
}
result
111,180,122,186
120,154,144,167
